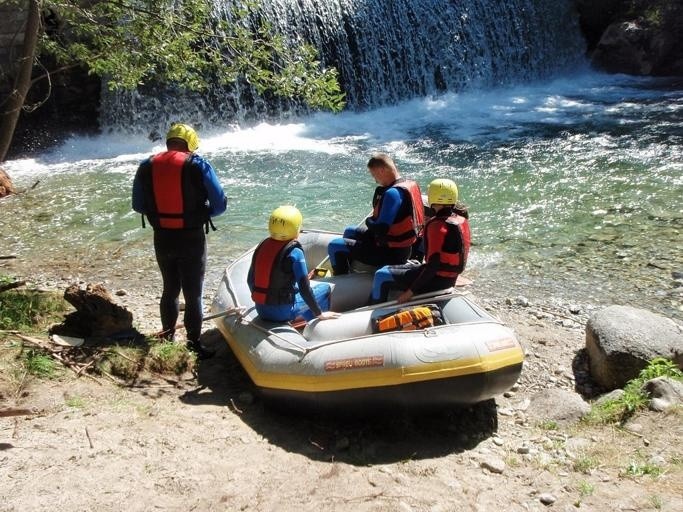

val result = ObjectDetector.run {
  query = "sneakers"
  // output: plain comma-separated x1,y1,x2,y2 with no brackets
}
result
186,338,216,359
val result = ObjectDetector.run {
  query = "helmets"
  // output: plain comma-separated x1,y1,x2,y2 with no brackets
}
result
428,179,458,209
166,124,199,152
269,206,303,241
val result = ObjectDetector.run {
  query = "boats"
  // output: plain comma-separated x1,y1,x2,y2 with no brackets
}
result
208,229,528,409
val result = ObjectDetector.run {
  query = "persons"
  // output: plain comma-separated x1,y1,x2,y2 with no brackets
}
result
328,153,425,276
247,206,341,324
132,124,228,351
368,178,470,306
